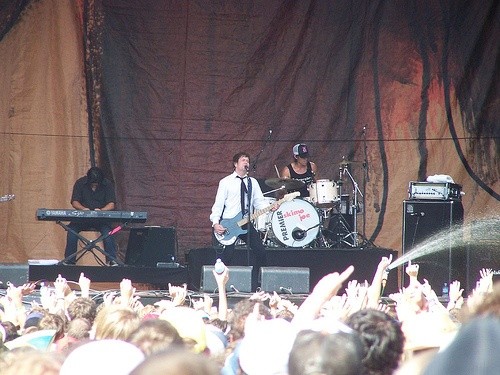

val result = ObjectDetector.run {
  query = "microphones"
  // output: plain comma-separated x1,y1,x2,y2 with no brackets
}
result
411,212,424,216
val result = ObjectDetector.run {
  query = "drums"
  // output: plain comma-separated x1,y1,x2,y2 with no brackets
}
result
308,178,340,209
251,196,278,231
270,198,320,247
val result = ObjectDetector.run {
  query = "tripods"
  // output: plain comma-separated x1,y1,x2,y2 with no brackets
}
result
261,165,364,248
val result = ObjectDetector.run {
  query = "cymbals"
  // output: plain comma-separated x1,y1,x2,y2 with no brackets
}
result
264,177,306,190
255,177,265,186
325,161,363,166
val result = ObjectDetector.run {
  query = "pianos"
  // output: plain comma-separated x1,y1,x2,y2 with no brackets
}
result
35,208,147,265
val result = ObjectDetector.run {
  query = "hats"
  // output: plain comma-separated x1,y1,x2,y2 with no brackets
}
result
293,143,310,157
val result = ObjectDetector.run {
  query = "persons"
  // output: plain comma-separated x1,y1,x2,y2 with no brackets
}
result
281,143,332,238
209,153,280,293
0,249,500,375
63,166,119,266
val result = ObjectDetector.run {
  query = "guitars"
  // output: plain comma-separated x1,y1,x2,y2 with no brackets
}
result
213,192,300,246
0,193,16,201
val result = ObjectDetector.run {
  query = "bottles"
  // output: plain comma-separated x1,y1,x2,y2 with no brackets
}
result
215,259,226,275
442,283,448,299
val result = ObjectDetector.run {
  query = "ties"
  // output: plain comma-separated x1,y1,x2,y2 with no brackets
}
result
236,176,248,219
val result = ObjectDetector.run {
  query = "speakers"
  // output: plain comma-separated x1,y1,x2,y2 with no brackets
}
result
400,200,465,305
258,266,310,295
125,227,177,265
201,264,252,293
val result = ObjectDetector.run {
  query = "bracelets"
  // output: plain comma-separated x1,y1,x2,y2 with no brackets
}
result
450,299,456,304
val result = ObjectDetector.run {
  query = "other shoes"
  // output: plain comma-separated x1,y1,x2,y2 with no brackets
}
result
63,259,76,266
105,258,118,267
